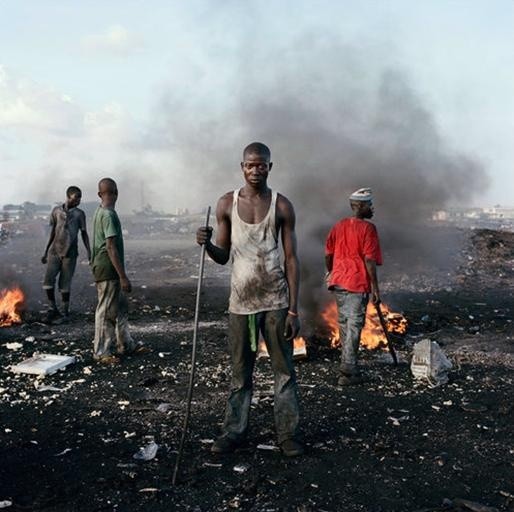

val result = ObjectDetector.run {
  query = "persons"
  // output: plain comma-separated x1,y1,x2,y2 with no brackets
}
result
325,188,383,388
196,143,304,458
90,178,151,364
41,186,91,318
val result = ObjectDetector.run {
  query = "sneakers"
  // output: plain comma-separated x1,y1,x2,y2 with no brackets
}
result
95,356,120,366
334,375,361,385
210,438,237,456
130,347,149,358
281,438,305,455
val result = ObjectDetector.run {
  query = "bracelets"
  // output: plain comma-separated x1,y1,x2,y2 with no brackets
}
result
288,311,298,317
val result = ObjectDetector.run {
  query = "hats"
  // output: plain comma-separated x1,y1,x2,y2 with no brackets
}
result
350,188,373,201
